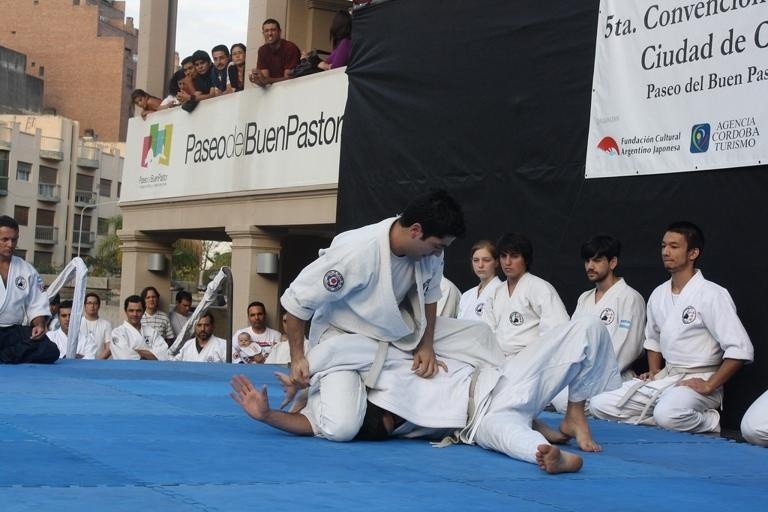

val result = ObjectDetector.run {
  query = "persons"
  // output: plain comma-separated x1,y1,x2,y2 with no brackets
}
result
43,286,310,364
591,220,753,432
546,231,645,416
741,390,768,448
1,215,60,364
229,187,621,474
131,9,351,120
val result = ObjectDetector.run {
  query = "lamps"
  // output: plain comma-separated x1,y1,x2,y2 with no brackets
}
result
148,253,167,272
256,252,278,274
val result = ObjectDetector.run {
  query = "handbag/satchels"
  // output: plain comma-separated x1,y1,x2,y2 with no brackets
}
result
287,54,323,78
